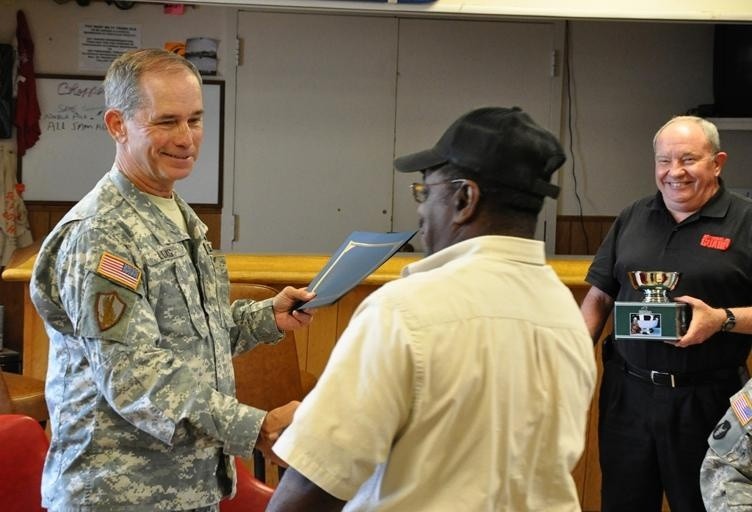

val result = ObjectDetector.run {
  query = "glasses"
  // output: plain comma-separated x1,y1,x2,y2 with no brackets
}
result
409,177,465,203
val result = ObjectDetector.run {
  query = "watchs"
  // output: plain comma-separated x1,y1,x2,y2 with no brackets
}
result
721,306,737,334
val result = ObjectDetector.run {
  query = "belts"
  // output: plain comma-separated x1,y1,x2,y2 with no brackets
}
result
624,368,695,390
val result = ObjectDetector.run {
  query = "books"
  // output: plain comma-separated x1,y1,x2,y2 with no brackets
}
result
291,226,421,312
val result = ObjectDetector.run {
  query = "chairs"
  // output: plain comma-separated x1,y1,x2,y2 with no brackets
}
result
229,282,321,485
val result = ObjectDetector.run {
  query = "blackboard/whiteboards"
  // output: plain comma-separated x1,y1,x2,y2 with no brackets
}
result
17,73,225,209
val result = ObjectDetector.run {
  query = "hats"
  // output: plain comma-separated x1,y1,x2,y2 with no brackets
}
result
393,104,567,199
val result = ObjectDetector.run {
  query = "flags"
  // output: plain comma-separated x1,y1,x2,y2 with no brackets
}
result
14,9,42,157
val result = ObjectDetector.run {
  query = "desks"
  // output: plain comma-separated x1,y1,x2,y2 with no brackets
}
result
1,250,671,512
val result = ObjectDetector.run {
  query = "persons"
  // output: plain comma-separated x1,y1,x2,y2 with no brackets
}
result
699,373,751,512
263,102,596,512
31,46,318,512
576,111,752,512
